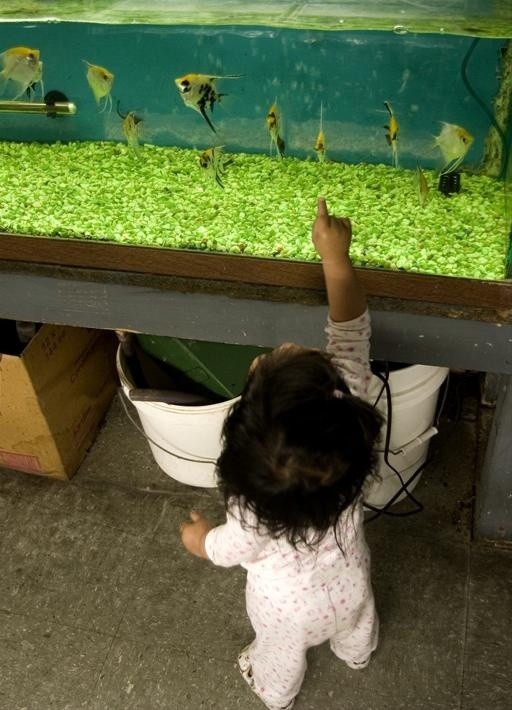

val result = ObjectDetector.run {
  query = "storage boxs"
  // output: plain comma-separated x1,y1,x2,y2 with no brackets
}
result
0,319,118,484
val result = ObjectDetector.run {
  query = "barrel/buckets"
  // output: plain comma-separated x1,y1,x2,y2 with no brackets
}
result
361,366,454,514
113,340,245,490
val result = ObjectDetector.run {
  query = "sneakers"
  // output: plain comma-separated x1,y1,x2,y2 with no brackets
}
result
238,644,294,709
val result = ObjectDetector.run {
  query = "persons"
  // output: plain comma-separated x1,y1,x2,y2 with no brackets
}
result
180,198,380,709
